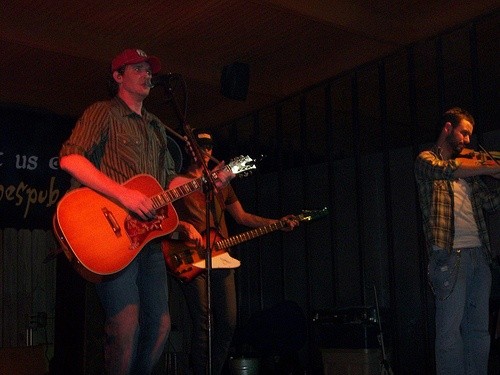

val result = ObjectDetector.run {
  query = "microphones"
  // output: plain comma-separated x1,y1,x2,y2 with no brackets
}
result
146,73,181,88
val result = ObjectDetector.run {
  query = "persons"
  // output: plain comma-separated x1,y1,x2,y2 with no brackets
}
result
58,47,237,375
414,107,500,375
163,127,301,375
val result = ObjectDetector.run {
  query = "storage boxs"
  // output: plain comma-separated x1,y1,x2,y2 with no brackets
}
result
320,348,394,375
319,325,379,349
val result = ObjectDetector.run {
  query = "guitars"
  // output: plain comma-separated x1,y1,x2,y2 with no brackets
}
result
162,207,331,281
52,154,257,282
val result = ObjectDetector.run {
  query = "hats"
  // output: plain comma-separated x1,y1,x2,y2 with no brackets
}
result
111,48,162,74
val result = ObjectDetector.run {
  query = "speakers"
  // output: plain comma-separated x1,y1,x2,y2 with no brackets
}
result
220,62,251,98
